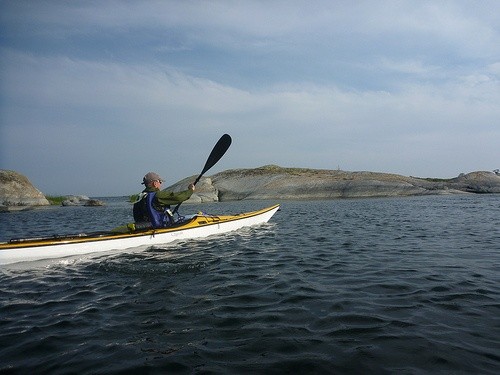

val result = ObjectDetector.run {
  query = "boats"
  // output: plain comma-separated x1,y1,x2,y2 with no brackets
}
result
1,201,280,267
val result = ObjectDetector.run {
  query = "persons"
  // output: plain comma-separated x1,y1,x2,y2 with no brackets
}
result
133,172,197,230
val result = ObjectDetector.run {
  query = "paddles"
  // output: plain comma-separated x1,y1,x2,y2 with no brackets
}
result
172,134,232,215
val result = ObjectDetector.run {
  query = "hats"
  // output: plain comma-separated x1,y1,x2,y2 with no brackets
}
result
141,172,168,185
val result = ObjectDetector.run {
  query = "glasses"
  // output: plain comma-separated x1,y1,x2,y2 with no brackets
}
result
157,180,162,184
